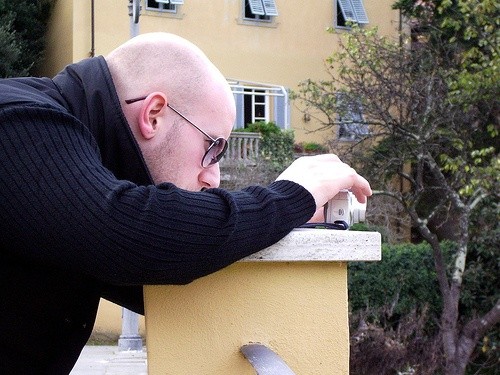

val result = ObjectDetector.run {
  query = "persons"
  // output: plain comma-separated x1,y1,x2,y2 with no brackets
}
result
0,31,373,375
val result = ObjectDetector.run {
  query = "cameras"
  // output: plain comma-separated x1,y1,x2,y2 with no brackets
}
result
324,189,367,229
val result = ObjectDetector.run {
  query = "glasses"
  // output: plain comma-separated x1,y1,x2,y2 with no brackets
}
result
125,95,229,169
